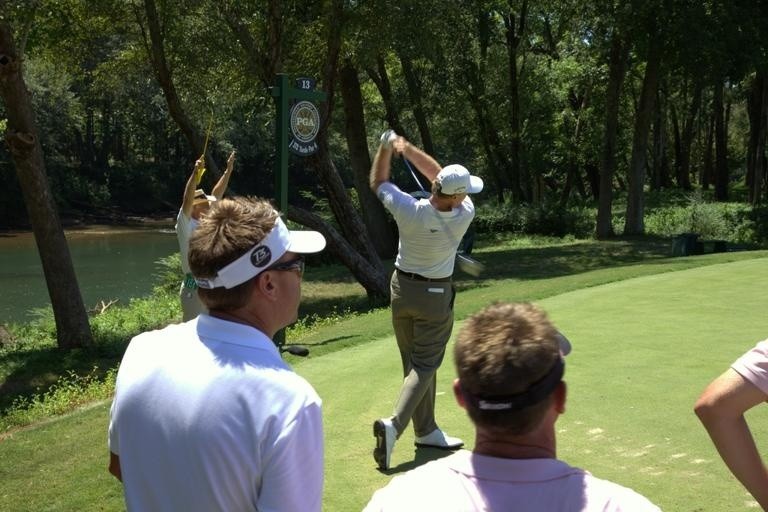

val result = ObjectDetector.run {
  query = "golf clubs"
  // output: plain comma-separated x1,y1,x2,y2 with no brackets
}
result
402,152,484,276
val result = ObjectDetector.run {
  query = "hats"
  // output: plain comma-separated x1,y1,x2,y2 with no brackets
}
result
192,189,217,206
459,330,572,414
194,210,327,290
435,164,484,198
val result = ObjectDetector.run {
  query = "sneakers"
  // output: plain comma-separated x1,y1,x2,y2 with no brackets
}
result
414,428,464,450
373,415,398,471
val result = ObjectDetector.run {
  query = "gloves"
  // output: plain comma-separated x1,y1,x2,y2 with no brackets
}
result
380,128,397,149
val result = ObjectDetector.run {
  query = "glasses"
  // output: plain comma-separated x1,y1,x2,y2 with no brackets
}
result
271,256,305,278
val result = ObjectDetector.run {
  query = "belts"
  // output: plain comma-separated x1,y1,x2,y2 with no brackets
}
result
394,267,453,283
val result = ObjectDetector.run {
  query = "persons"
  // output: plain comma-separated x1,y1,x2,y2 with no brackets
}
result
106,191,334,512
357,299,665,512
689,334,768,511
175,150,236,324
366,129,486,472
